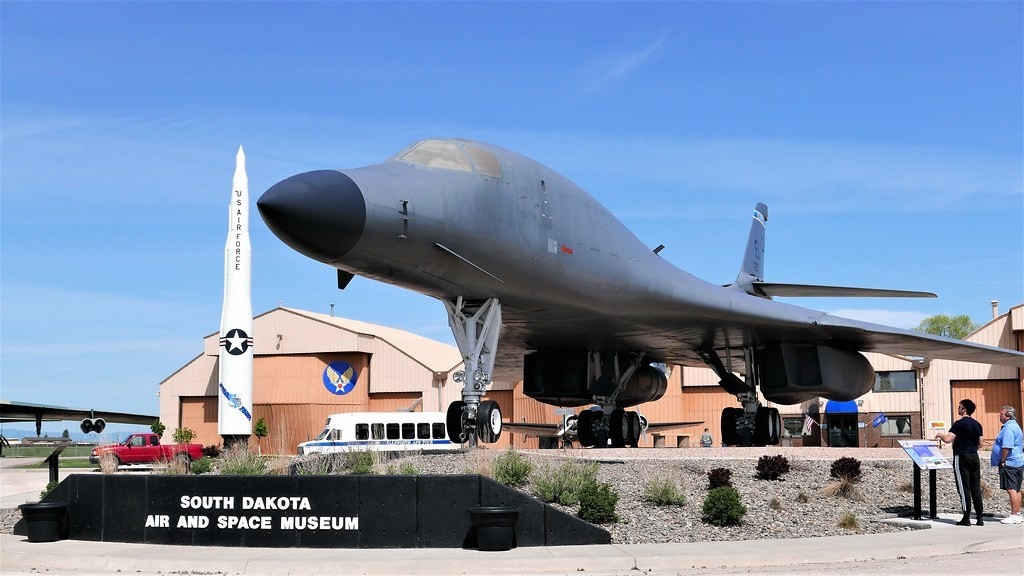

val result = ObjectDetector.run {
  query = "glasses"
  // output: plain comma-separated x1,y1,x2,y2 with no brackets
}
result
959,405,964,409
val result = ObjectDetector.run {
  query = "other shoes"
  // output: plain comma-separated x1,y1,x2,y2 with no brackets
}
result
955,517,971,526
977,520,983,526
1017,512,1024,521
1001,515,1021,524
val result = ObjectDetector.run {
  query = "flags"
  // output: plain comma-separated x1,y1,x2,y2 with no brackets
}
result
873,414,885,428
806,415,814,432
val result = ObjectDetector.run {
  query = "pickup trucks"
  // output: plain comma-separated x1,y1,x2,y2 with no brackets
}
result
88,433,207,473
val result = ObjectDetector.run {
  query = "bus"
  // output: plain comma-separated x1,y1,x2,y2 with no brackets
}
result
295,410,461,459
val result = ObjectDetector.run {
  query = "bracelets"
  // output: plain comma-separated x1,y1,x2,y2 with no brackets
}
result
1000,462,1005,463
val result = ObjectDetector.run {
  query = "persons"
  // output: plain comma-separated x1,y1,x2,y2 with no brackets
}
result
991,406,1024,524
701,428,713,447
935,399,984,526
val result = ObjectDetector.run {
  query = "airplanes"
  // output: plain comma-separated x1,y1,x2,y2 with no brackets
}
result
254,136,1023,450
498,398,706,447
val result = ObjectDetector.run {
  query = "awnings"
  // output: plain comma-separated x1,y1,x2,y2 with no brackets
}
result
825,400,858,413
